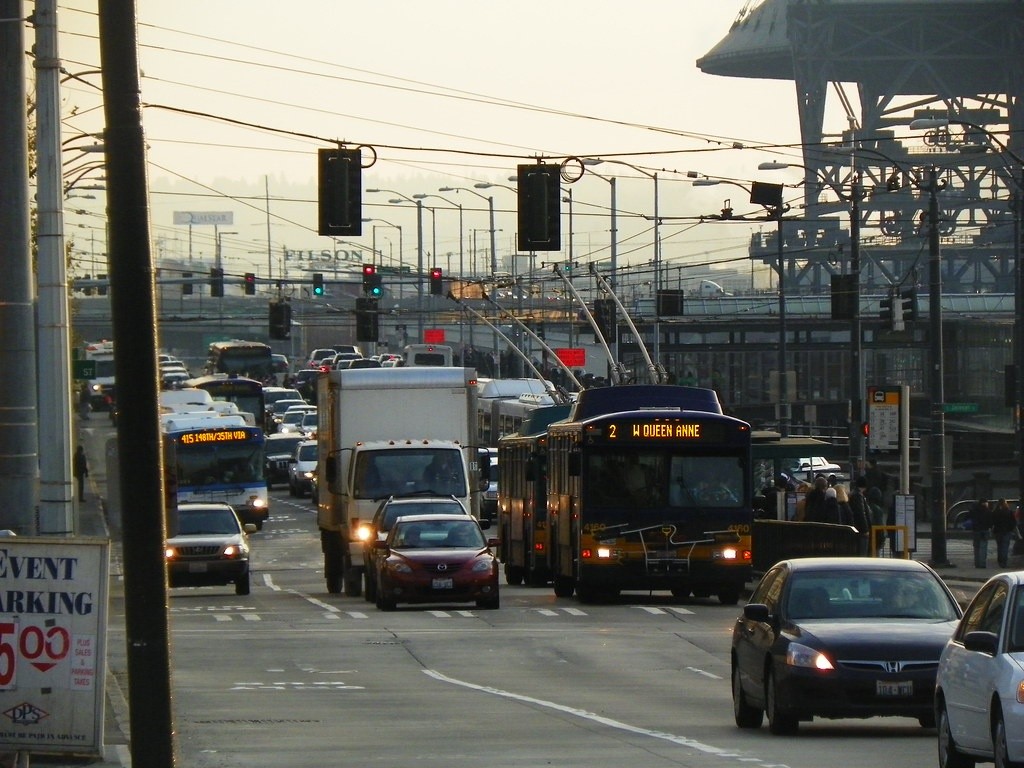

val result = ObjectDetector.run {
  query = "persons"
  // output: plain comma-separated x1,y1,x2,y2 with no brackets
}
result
419,455,455,496
480,476,489,490
806,587,832,618
536,462,737,506
990,497,1015,567
760,468,900,562
972,499,992,569
880,582,920,612
227,453,255,485
73,445,88,503
79,382,93,420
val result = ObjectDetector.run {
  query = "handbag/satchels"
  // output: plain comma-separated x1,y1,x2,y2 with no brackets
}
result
1012,526,1022,541
962,514,976,527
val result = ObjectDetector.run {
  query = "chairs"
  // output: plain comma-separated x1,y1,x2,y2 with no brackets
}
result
442,525,468,543
865,585,914,615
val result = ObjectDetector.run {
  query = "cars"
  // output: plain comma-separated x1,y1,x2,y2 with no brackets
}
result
933,570,1023,766
262,386,318,498
374,514,500,609
731,555,965,734
479,447,499,513
272,345,403,386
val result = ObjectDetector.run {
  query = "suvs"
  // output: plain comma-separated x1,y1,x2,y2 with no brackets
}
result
165,501,260,593
361,495,492,605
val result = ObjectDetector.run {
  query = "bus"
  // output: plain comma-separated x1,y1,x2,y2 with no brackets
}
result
545,385,755,603
498,405,570,587
78,341,276,526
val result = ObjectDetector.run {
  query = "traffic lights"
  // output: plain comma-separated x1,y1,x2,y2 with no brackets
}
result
431,268,442,296
313,273,323,295
245,274,255,295
878,298,894,328
363,265,383,298
901,289,919,321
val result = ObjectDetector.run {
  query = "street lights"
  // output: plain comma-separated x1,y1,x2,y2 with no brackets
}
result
363,217,403,322
368,188,435,329
825,145,950,565
337,242,382,266
693,177,788,437
758,159,866,483
909,118,1023,564
389,157,662,385
217,229,239,267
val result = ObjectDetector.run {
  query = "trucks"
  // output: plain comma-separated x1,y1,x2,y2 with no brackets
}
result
476,374,557,448
315,367,491,594
403,344,452,368
